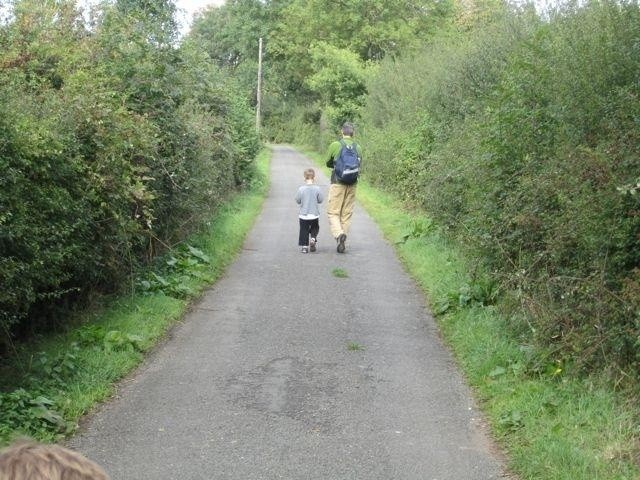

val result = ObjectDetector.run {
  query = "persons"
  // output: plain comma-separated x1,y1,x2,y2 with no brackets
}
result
324,122,364,254
292,169,324,254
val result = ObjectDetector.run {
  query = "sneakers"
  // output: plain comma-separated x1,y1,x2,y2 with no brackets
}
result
302,248,308,253
310,239,315,252
337,234,346,253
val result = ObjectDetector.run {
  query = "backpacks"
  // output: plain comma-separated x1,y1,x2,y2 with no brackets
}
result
335,138,360,184
